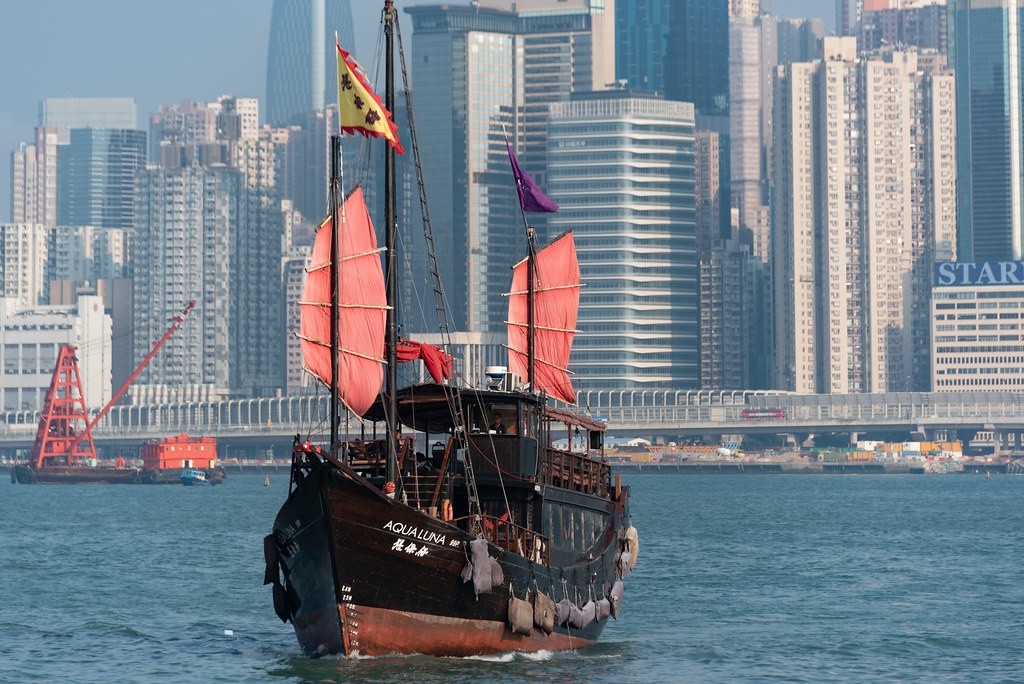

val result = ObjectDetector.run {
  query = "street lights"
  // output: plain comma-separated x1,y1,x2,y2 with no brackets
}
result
223,443,231,459
271,444,275,460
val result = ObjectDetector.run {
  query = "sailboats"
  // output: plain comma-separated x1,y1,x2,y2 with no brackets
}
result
261,2,641,664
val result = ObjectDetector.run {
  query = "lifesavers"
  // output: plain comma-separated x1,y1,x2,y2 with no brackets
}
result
442,499,454,522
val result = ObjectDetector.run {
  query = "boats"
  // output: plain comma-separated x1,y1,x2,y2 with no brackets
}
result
179,469,209,486
263,472,270,486
7,462,140,485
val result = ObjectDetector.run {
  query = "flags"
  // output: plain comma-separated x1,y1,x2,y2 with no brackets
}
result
506,138,560,213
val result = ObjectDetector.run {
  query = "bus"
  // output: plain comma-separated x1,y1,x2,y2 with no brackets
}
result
739,407,785,422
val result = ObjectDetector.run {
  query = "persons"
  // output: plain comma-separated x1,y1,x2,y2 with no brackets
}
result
490,413,507,435
